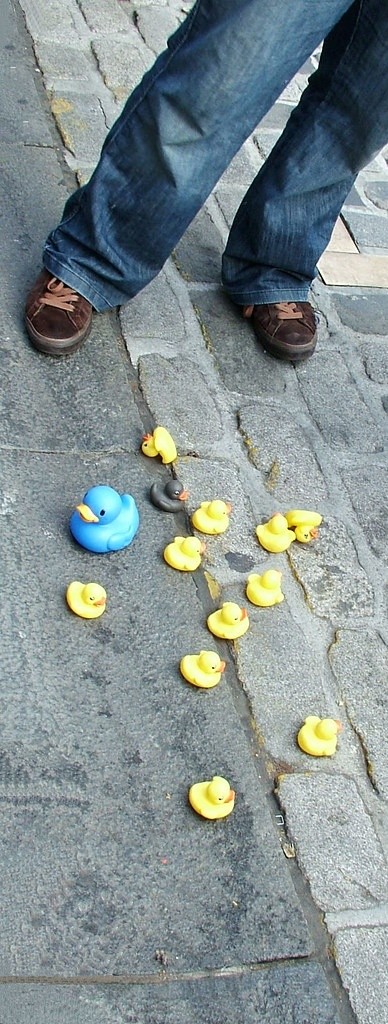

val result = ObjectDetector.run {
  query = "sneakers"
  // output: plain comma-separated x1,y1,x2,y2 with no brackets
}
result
26,267,93,356
244,301,317,361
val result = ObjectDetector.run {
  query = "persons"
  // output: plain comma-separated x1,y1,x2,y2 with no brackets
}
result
24,0,388,359
